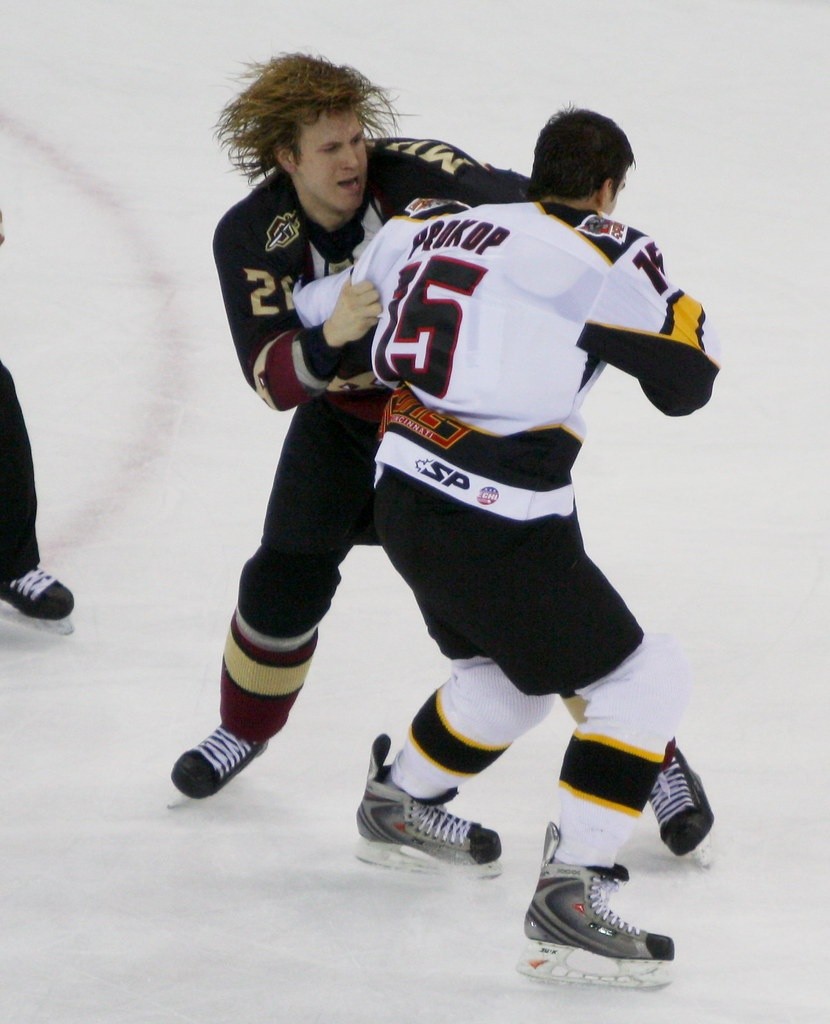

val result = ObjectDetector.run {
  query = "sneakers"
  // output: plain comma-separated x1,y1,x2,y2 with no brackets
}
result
355,734,503,878
515,822,674,989
166,725,269,808
1,567,76,635
647,747,714,869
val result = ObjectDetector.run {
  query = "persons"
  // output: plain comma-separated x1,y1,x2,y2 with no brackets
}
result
0,207,75,636
170,52,716,872
294,109,722,989
